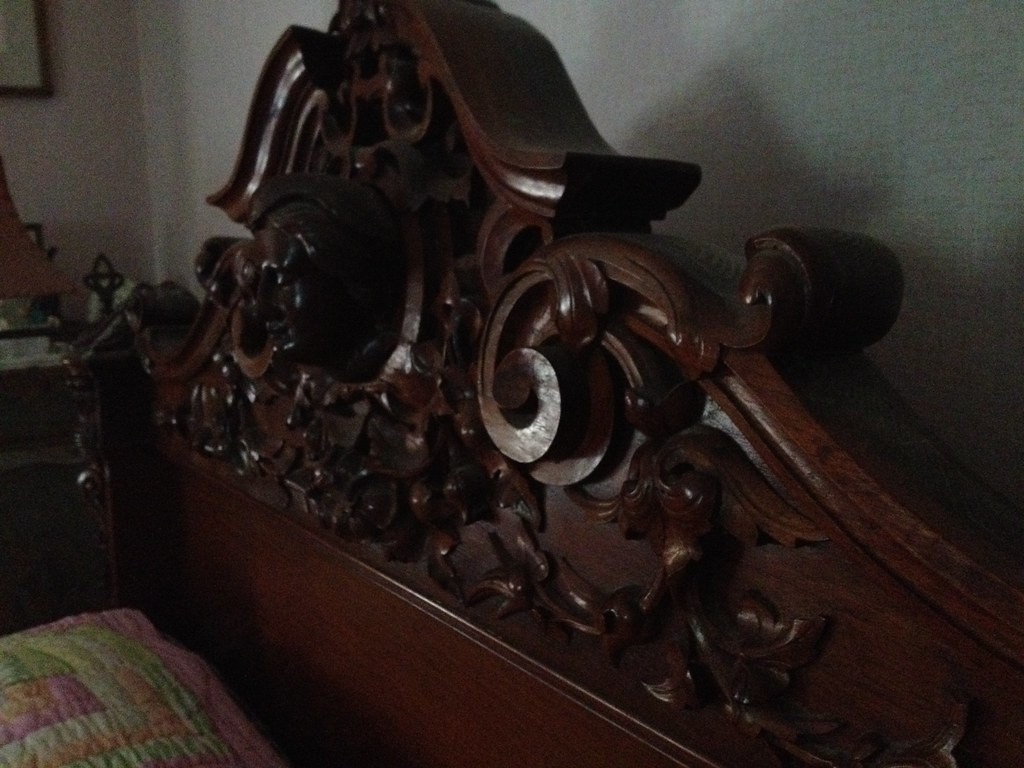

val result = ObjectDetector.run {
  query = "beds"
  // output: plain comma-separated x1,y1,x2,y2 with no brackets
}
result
64,0,1024,768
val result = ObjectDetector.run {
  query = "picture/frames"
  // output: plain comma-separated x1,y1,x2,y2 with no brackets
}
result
0,0,53,98
0,224,60,340
